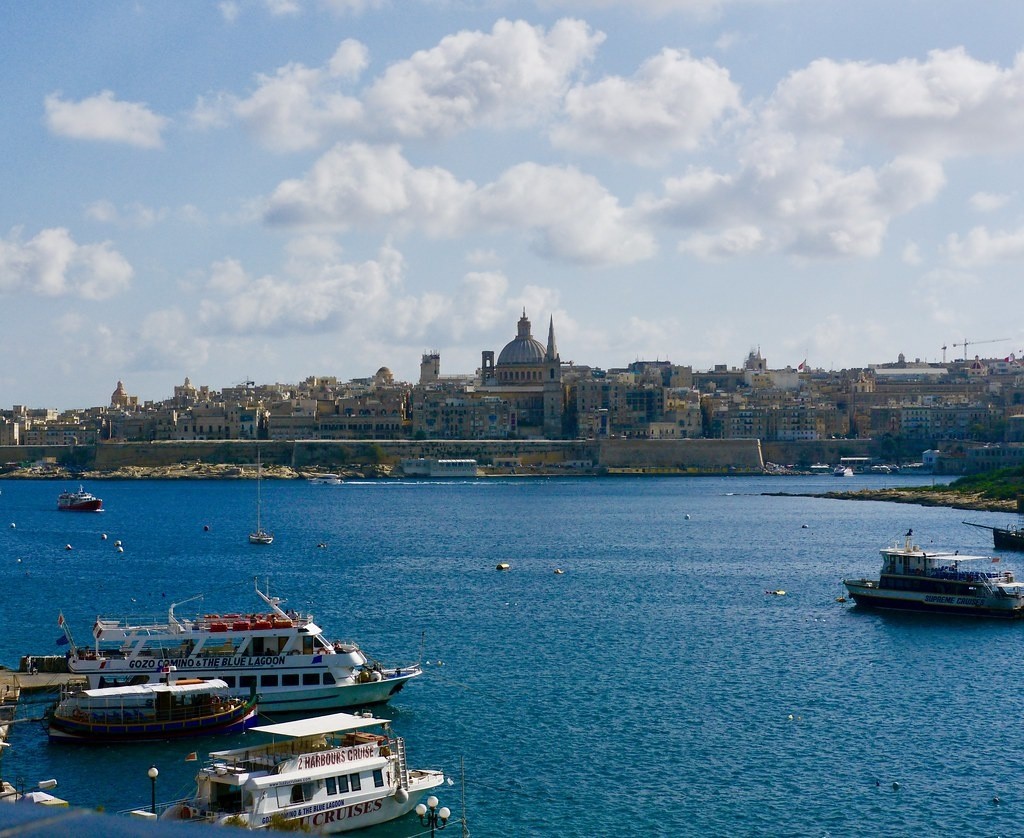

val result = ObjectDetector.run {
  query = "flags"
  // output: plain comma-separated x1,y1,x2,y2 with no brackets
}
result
56,635,69,646
185,752,198,762
58,615,64,627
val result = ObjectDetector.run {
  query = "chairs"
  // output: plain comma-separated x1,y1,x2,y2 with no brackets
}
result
908,566,997,582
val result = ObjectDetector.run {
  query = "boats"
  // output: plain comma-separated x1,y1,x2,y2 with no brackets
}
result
40,666,262,744
838,526,1024,620
961,519,1024,550
57,484,103,511
833,464,855,477
116,709,447,836
58,576,426,713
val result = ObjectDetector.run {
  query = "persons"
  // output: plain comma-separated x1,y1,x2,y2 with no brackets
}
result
25,608,382,723
912,564,974,582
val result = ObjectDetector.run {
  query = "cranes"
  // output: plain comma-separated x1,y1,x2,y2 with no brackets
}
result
953,337,1013,360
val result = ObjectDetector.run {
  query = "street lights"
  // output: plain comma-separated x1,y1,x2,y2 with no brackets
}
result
415,796,451,838
148,764,158,813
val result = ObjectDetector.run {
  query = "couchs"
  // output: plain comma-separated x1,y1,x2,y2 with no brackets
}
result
205,613,293,629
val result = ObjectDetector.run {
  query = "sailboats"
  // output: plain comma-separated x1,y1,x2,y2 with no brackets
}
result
247,448,275,544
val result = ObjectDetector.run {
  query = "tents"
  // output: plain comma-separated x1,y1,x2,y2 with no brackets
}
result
75,678,229,721
249,713,392,754
